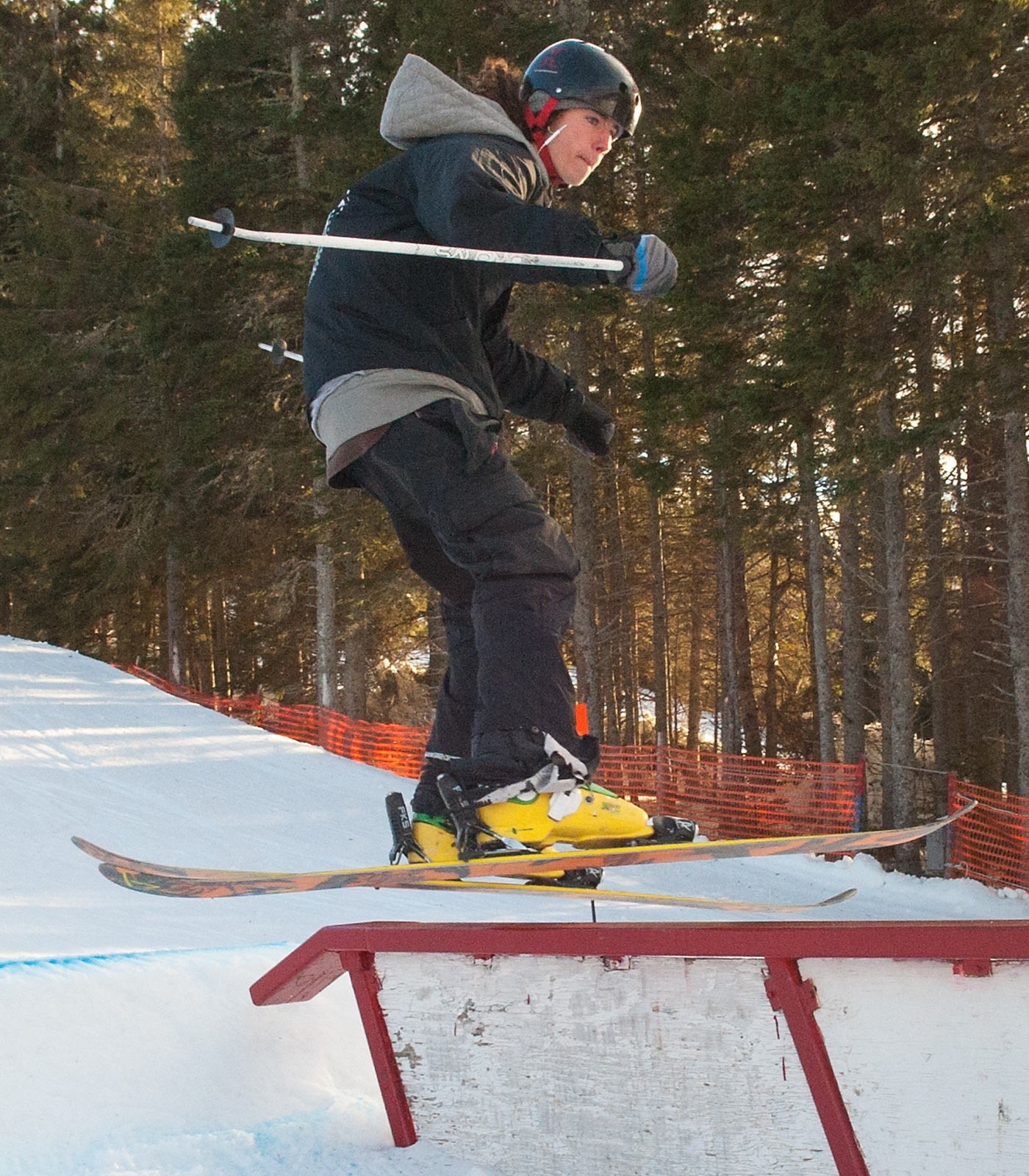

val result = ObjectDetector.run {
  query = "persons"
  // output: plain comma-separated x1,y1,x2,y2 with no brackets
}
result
301,36,680,888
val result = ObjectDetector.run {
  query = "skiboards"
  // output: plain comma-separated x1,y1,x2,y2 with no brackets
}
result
71,795,982,912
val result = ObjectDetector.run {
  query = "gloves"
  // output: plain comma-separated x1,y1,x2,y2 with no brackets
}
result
597,233,679,297
558,390,616,459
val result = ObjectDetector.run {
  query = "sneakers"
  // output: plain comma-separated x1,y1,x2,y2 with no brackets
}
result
408,813,565,880
474,779,655,858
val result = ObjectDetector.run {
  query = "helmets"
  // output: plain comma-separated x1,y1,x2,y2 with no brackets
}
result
517,38,642,141
